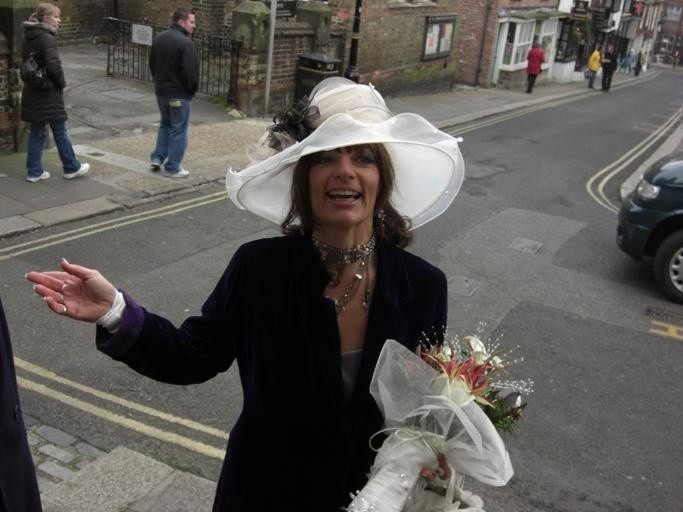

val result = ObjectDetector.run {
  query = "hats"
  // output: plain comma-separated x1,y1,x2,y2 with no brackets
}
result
226,77,465,232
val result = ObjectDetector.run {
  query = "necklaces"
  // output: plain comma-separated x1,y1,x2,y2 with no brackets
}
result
310,226,376,311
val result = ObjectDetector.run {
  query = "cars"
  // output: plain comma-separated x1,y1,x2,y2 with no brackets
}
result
614,151,682,305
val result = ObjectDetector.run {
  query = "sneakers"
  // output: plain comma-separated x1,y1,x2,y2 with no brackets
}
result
151,162,189,178
64,163,90,179
26,171,50,182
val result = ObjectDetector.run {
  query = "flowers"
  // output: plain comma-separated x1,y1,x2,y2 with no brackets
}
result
344,322,533,510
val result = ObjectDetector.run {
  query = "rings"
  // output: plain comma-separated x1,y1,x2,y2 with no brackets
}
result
62,306,66,313
59,294,63,301
60,281,68,291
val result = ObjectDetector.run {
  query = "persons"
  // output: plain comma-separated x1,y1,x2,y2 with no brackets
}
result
633,50,645,78
21,77,466,511
601,47,617,92
0,293,46,510
147,8,200,177
622,51,634,76
524,42,544,94
586,45,606,89
671,50,680,72
19,2,90,183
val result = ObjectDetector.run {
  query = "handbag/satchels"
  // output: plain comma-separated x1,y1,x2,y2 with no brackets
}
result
584,71,593,78
19,56,53,91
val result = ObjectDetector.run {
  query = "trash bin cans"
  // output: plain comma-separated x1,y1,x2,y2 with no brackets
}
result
294,52,342,103
